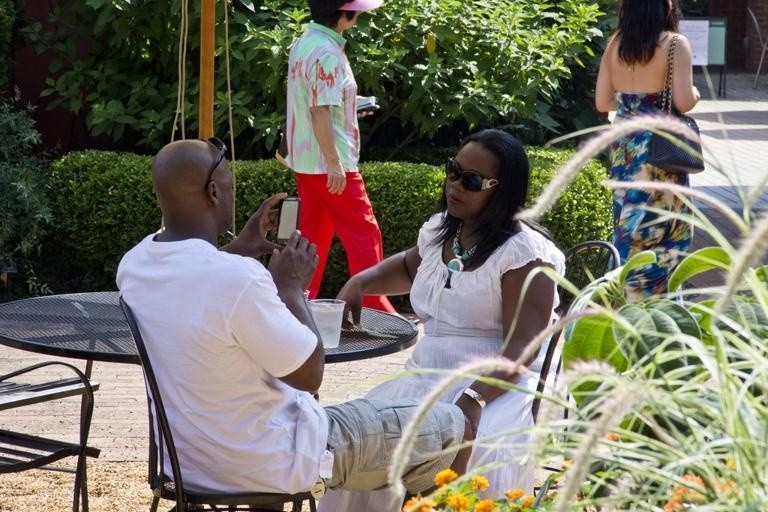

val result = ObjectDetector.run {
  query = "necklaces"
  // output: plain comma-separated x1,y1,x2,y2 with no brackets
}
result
446,223,478,281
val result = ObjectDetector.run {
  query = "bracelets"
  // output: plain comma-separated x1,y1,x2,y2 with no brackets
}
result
463,388,487,407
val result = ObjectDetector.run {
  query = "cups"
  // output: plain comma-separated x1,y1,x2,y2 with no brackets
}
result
307,298,347,351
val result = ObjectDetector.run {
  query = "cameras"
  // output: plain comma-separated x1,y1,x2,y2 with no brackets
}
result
356,95,380,111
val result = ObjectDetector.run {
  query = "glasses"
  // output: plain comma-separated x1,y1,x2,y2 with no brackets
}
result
206,136,228,187
446,155,491,192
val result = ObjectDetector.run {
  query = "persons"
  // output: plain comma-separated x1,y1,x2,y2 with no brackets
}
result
116,135,474,512
594,0,700,303
285,1,399,314
317,129,577,512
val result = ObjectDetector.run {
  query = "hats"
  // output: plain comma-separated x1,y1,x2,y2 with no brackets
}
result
339,0,383,11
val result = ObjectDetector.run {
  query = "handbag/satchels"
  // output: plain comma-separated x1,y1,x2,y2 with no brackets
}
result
645,36,705,175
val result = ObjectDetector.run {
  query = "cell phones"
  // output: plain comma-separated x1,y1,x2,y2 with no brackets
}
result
276,198,301,246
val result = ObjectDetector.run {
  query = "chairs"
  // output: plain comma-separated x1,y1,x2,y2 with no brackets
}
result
0,361,99,511
531,241,622,512
120,297,317,512
